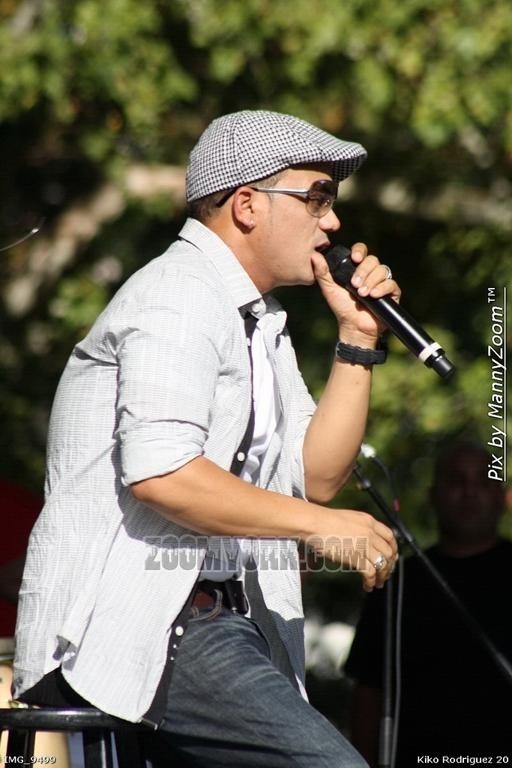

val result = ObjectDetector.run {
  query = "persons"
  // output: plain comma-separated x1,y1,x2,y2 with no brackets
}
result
12,106,402,768
338,441,512,768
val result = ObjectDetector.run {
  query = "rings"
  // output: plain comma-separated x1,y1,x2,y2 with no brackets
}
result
384,264,393,279
373,554,385,570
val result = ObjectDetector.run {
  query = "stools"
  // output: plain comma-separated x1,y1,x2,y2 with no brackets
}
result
0,707,122,768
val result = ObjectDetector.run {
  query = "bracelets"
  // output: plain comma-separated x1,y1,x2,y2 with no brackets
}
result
338,341,386,365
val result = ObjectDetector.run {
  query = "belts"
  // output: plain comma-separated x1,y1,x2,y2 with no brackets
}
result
191,581,247,613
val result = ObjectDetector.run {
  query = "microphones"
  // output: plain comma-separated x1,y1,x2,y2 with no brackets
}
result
326,247,455,378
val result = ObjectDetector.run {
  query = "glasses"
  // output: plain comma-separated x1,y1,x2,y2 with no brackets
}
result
217,180,338,218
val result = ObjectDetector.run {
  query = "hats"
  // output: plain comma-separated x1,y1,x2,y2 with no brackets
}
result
186,110,368,203
